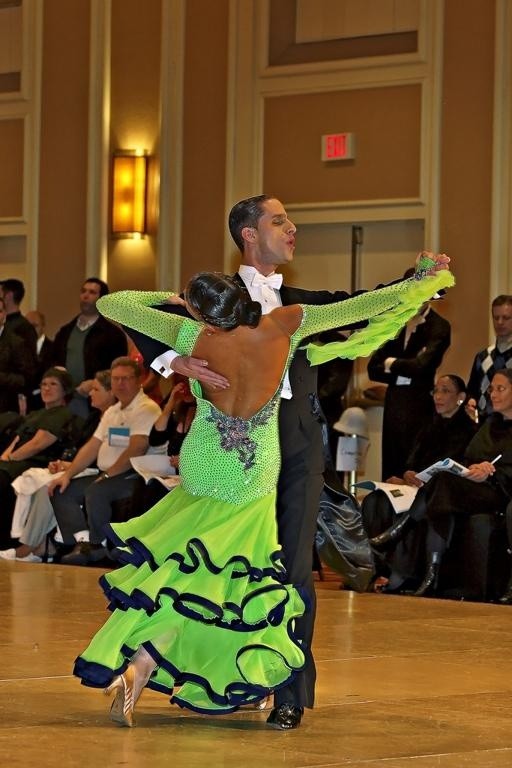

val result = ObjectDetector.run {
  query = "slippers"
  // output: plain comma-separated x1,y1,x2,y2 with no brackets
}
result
15,552,54,563
0,548,33,561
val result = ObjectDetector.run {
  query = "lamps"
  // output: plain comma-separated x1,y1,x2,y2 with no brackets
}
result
110,147,148,240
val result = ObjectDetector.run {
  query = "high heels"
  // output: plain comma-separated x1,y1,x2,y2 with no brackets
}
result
413,551,441,596
368,511,416,553
103,674,138,729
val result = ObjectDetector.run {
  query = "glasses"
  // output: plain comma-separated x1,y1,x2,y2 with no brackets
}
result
486,386,510,395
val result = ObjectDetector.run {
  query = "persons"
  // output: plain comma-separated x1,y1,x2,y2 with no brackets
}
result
118,191,453,729
310,271,511,604
2,278,197,571
69,249,456,729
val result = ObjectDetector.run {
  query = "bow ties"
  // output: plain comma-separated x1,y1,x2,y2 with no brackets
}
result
250,272,284,293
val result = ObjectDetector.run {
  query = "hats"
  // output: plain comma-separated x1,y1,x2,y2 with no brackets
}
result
332,406,370,442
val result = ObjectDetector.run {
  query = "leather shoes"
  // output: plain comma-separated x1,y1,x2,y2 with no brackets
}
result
489,585,511,603
81,547,117,571
61,541,91,567
378,577,408,595
265,705,305,730
